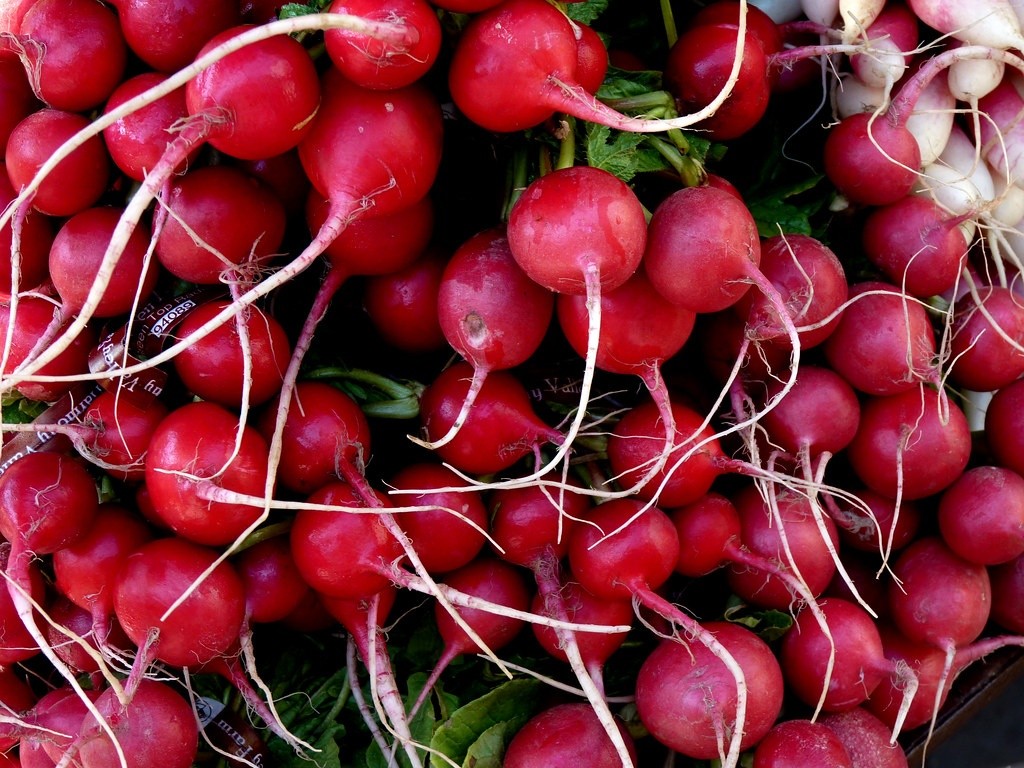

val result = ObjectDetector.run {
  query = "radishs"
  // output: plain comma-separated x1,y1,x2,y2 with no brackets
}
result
797,0,1024,288
